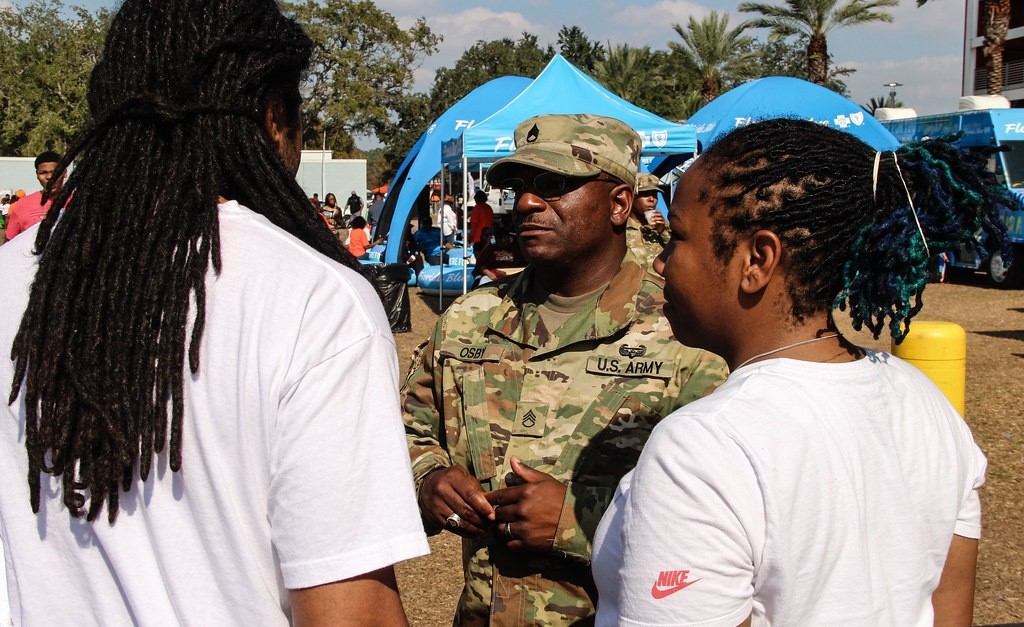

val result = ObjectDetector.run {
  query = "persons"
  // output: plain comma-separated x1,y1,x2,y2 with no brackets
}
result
0,0,430,627
0,151,73,244
399,114,733,627
310,185,386,256
405,189,524,282
582,120,1024,627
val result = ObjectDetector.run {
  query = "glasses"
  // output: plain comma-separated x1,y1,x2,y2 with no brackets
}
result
500,172,622,196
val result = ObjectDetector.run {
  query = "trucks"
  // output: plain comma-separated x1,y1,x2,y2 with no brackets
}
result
875,108,1024,289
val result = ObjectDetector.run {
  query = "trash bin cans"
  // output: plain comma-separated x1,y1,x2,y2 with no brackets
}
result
360,264,412,332
890,321,966,420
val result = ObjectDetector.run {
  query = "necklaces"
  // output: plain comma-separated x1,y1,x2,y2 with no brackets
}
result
737,331,844,368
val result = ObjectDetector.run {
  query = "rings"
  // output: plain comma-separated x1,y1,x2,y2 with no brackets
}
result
447,514,461,528
504,522,515,539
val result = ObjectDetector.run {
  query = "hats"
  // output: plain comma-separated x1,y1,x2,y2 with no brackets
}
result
486,115,641,193
637,173,664,192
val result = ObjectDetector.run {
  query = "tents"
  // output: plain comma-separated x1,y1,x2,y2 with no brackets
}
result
373,76,533,262
651,75,899,210
438,51,700,311
